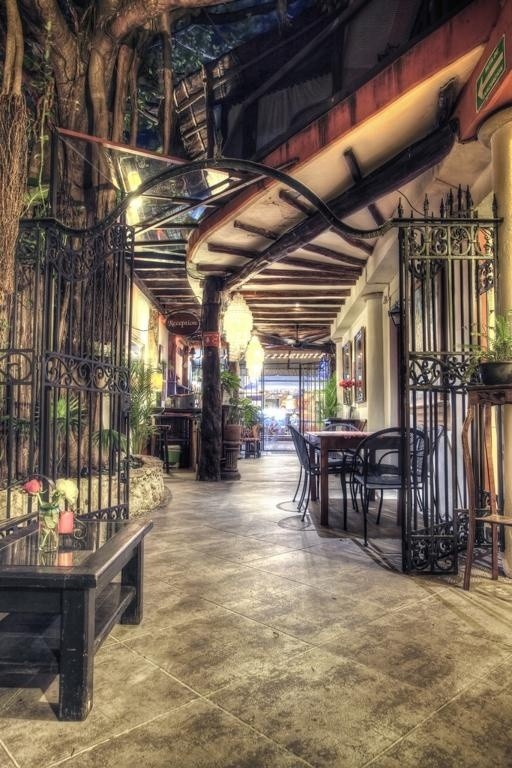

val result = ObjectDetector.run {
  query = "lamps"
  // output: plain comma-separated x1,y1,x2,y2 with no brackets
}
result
148,366,163,392
386,298,407,327
244,332,265,384
222,280,253,364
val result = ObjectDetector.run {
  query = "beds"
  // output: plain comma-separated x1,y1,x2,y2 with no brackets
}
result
1,517,155,721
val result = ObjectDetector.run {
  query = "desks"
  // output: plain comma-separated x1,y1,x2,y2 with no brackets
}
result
146,405,201,472
457,383,511,590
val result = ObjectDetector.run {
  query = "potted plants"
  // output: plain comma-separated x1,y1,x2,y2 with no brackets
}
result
458,305,512,384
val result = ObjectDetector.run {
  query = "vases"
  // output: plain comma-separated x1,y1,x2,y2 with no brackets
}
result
343,392,350,404
36,504,60,553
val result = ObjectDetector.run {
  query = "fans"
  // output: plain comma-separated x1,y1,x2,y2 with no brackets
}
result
252,323,333,351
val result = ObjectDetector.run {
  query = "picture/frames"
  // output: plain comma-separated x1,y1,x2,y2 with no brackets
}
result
342,340,352,404
354,327,367,404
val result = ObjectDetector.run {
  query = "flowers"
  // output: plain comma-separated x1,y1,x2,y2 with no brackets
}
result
22,473,80,548
340,380,354,405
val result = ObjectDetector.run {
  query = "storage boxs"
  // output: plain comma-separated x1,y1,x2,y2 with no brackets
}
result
162,444,181,469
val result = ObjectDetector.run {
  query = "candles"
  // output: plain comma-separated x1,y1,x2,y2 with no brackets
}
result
59,511,74,534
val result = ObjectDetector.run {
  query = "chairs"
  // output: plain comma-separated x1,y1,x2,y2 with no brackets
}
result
285,417,438,546
239,423,261,458
